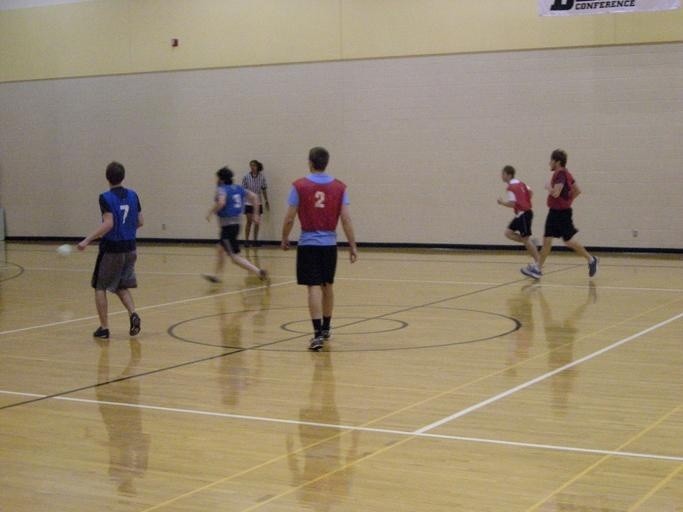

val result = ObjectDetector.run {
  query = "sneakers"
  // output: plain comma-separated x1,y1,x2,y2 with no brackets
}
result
309,336,324,350
588,255,598,278
520,237,545,279
259,269,272,286
129,313,141,336
322,330,330,341
202,273,221,284
93,326,109,339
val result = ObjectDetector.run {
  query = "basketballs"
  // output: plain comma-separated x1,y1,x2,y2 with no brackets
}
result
57,247,69,256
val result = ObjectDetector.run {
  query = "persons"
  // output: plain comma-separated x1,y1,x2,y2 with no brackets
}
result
496,166,540,262
202,167,272,285
76,160,144,339
522,149,597,280
241,160,270,247
282,146,358,349
